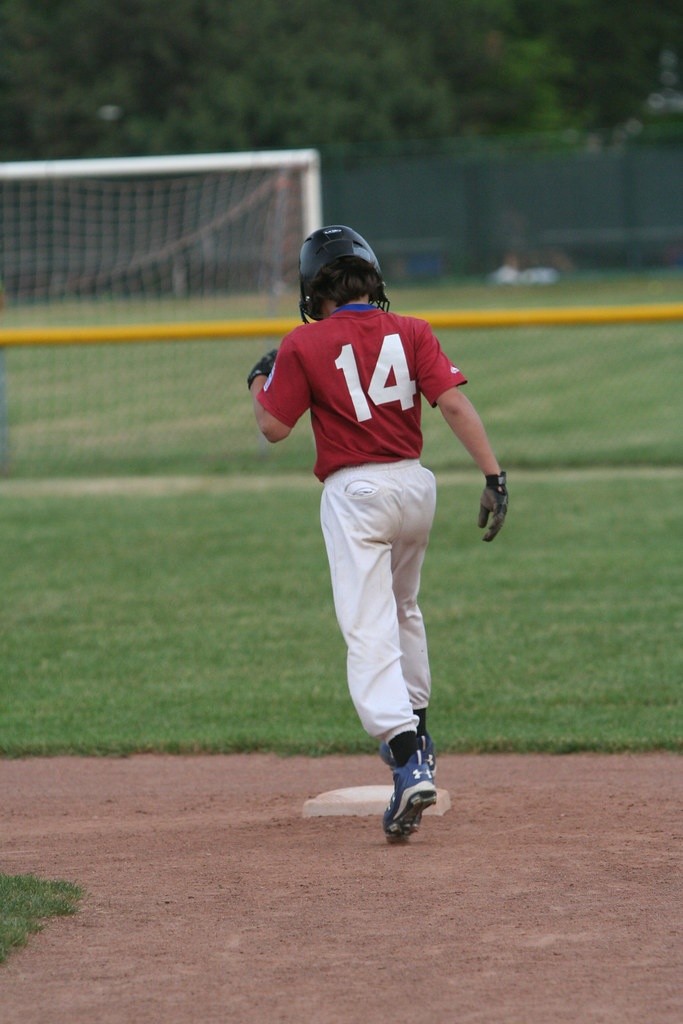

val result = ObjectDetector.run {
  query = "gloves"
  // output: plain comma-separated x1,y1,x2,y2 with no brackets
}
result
478,471,508,542
247,349,278,389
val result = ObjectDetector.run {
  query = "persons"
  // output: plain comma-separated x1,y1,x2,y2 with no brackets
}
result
247,227,508,836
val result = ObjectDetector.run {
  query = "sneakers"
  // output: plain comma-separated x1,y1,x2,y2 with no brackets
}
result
382,749,437,843
379,734,437,777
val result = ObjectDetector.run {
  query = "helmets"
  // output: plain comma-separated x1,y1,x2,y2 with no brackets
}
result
298,225,391,320
